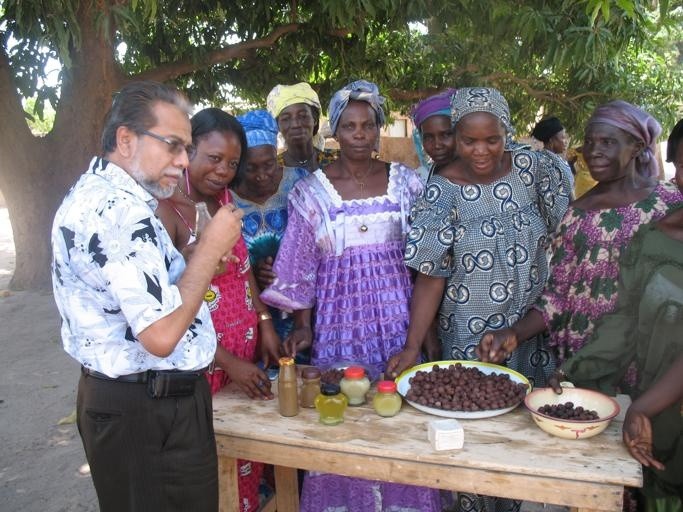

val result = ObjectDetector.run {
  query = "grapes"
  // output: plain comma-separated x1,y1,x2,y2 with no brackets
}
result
538,179,682,393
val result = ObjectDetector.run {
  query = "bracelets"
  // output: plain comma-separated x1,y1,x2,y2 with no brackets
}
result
258,313,272,321
555,369,568,377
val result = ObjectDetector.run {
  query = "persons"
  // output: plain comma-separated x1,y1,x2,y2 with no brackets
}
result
228,110,316,493
409,88,458,190
385,87,576,512
474,98,683,512
623,351,683,472
548,118,683,511
258,77,442,511
50,80,245,512
155,109,283,512
530,115,600,201
265,82,335,211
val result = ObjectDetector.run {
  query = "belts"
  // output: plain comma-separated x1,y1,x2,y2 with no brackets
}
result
83,368,153,383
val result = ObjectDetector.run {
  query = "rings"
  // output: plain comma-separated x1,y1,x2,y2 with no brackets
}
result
240,217,246,227
256,381,262,388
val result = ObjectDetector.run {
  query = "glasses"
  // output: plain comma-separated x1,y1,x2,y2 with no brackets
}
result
135,128,197,161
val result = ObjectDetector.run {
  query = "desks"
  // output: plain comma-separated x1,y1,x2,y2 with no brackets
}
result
213,373,643,511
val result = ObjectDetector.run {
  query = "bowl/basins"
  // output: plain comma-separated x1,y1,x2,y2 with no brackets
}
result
524,384,621,441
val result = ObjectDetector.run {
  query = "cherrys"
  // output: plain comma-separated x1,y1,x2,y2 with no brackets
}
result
403,362,529,413
537,401,600,421
318,366,344,383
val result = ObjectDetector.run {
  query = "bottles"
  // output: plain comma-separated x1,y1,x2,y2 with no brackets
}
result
278,356,402,427
194,201,226,275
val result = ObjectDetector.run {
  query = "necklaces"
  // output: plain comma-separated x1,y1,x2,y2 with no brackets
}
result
286,148,314,164
286,148,317,165
177,182,216,210
348,161,372,192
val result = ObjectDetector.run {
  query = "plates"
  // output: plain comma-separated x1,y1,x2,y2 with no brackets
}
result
394,359,532,419
